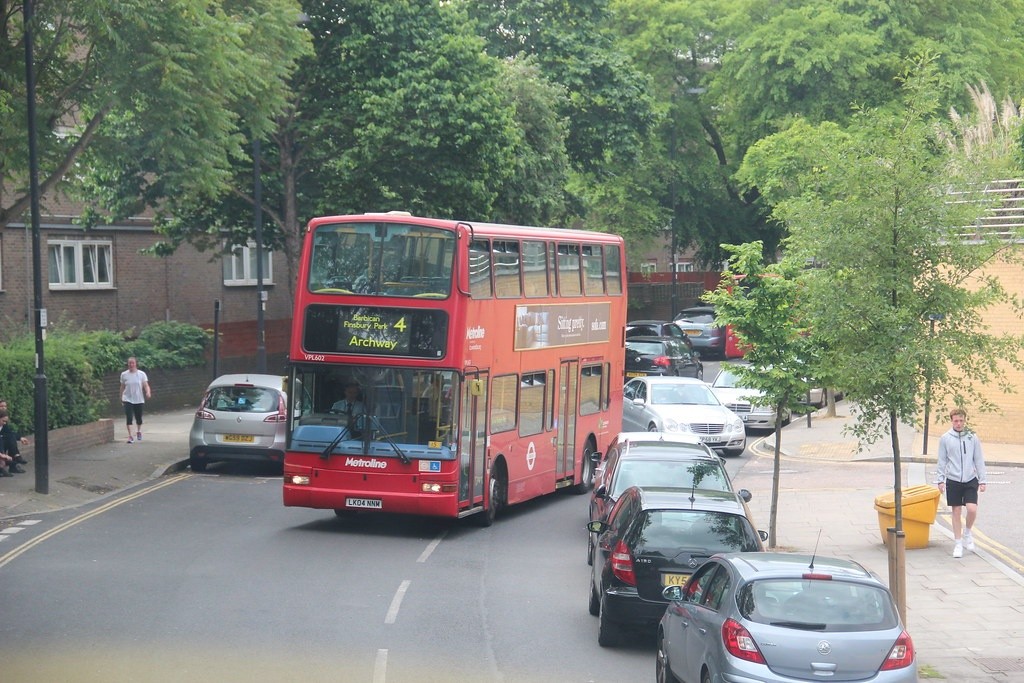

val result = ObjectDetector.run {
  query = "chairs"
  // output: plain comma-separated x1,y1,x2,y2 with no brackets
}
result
643,512,674,538
754,585,778,606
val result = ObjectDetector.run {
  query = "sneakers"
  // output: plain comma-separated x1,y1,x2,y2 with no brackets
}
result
137,431,142,440
127,436,134,443
962,528,974,549
953,543,963,557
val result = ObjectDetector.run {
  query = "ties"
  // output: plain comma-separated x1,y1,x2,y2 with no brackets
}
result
346,403,352,421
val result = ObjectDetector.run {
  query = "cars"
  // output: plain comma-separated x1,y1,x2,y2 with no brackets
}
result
586,438,753,567
671,304,725,362
777,351,844,407
189,372,312,473
586,486,770,647
623,375,746,456
591,430,727,493
625,319,701,360
625,336,704,381
655,550,916,683
712,361,792,435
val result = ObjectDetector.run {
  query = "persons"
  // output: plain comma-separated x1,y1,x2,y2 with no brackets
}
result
0,400,29,477
421,374,452,398
119,356,151,443
331,382,368,438
936,409,988,558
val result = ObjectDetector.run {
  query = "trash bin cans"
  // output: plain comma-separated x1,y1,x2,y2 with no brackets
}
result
873,485,940,549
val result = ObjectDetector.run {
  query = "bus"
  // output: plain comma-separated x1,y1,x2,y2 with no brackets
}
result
723,272,815,359
280,209,629,527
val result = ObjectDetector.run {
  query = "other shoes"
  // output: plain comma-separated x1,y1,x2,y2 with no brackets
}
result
13,455,28,464
9,464,26,473
0,468,14,476
